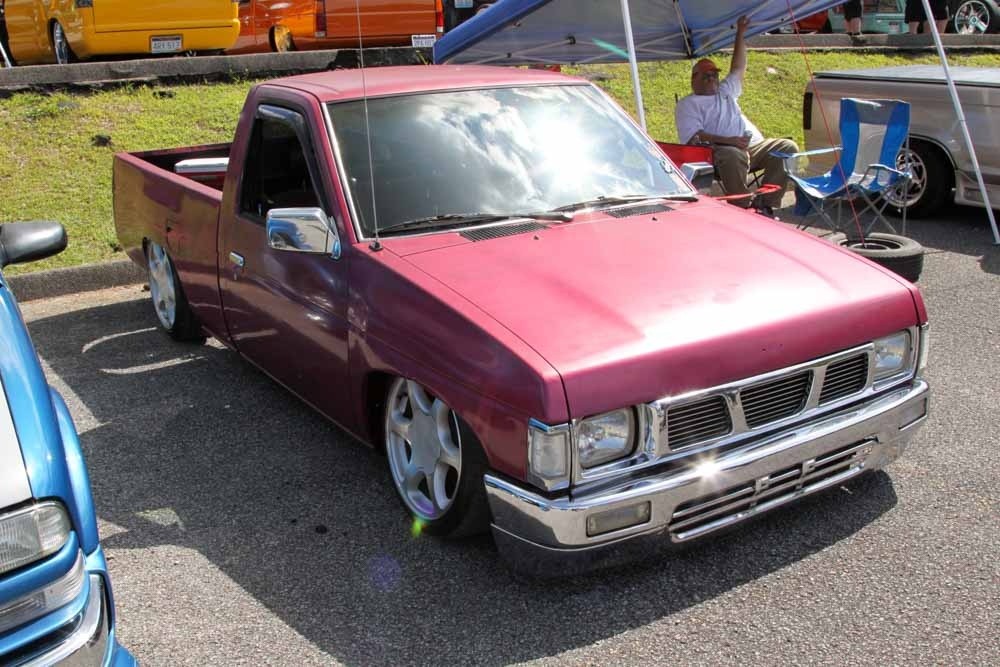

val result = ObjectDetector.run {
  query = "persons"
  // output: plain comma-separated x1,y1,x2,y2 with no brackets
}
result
675,14,799,218
904,0,949,34
842,0,863,36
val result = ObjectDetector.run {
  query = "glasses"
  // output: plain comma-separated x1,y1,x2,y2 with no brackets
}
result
695,72,719,80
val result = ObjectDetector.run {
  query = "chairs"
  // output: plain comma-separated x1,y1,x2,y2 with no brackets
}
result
256,136,317,224
644,93,792,217
768,97,914,241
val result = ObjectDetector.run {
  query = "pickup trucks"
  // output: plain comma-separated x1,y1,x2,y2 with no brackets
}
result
802,63,1000,223
111,66,932,584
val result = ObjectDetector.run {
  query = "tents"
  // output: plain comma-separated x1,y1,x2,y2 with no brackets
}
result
433,0,1000,243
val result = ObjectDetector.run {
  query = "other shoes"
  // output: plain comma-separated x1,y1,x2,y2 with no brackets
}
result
758,206,784,221
846,34,864,37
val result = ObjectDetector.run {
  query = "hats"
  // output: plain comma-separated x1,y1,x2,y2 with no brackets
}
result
693,59,721,74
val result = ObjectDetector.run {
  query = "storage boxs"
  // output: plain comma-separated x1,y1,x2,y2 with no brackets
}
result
175,157,230,193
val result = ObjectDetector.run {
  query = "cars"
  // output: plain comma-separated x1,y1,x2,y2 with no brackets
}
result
795,1,1000,56
224,0,445,54
0,0,240,67
0,218,140,667
